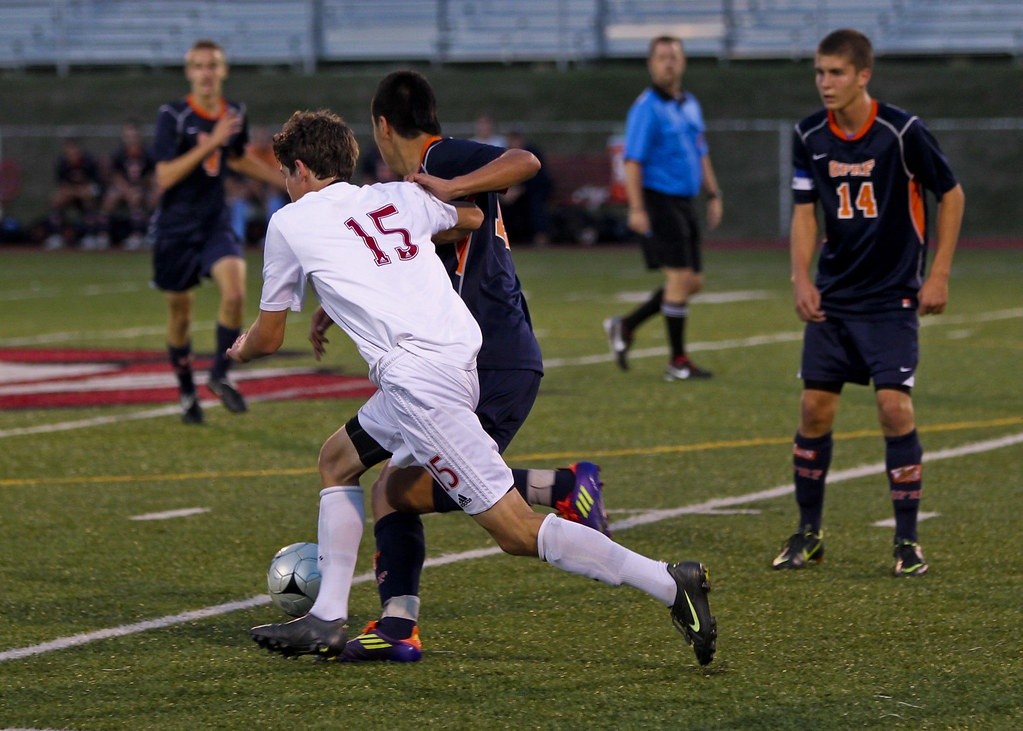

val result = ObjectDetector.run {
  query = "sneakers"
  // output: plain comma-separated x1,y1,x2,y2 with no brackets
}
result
663,356,712,383
335,620,422,663
771,524,825,571
667,560,717,666
603,318,633,373
249,612,348,659
556,462,610,538
893,536,928,579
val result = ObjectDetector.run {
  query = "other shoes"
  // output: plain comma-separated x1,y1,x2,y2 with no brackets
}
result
207,369,246,414
97,232,110,250
48,235,66,252
125,233,142,251
81,234,96,250
178,384,202,423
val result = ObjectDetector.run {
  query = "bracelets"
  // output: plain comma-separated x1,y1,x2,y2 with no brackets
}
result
706,189,720,199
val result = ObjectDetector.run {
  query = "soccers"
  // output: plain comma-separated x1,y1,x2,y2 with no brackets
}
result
267,542,324,619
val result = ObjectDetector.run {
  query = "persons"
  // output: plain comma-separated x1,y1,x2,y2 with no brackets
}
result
771,30,966,576
224,73,717,663
38,113,636,246
314,75,616,660
146,38,289,424
601,35,722,382
